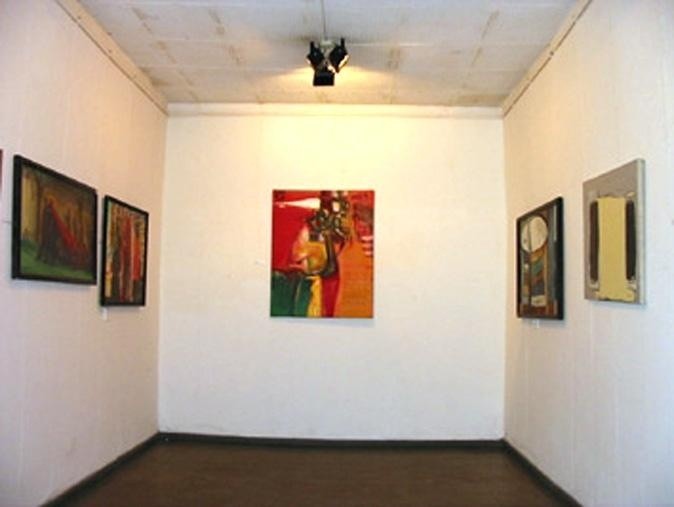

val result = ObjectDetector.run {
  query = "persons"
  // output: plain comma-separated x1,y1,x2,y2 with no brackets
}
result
37,192,84,265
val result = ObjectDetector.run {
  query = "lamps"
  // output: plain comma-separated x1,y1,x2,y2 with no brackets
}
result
304,32,352,87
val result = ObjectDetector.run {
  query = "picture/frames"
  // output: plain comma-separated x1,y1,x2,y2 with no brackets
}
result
10,153,98,286
512,195,569,321
97,192,149,308
